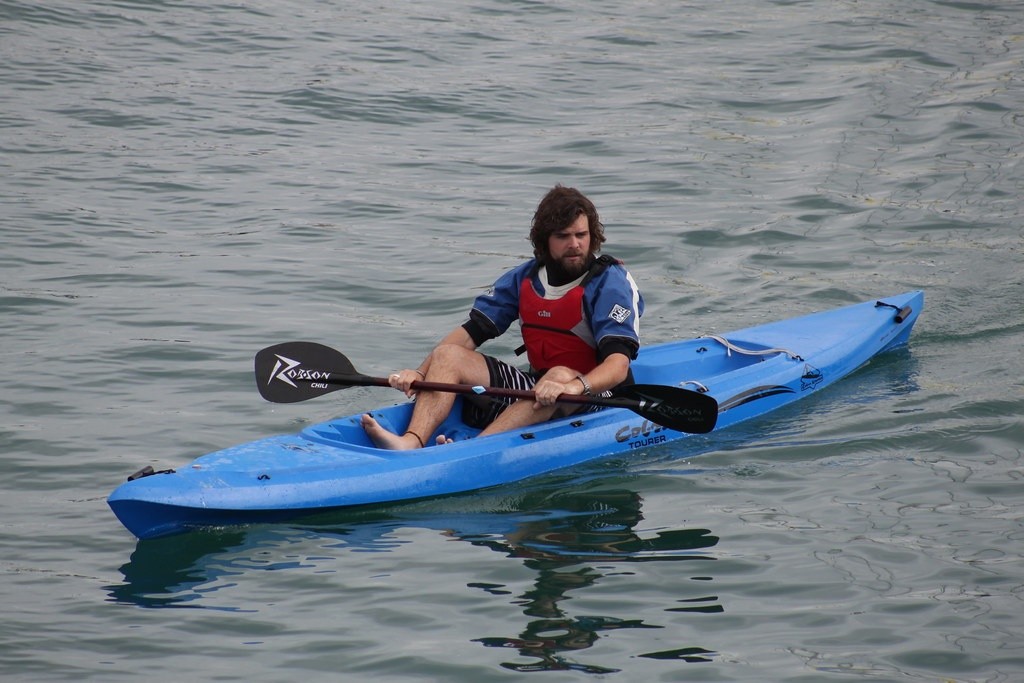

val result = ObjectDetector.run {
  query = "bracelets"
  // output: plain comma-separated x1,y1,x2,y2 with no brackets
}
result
416,367,427,378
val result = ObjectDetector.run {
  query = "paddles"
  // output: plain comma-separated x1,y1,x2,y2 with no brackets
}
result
249,340,719,437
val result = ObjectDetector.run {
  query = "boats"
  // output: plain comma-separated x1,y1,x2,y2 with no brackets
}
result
106,290,925,541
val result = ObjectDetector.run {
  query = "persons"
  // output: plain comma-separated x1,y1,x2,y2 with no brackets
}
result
362,185,645,459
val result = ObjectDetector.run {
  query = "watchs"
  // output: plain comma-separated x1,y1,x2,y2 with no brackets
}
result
573,374,592,397
